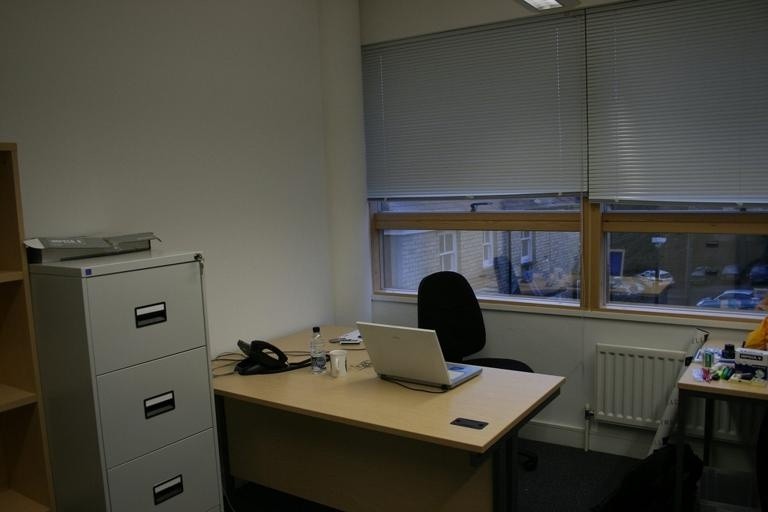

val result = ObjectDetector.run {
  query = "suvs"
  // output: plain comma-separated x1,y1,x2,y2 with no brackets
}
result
637,268,673,286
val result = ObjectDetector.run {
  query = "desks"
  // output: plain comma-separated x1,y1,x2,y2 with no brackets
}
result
209,323,566,511
675,340,768,467
488,276,671,304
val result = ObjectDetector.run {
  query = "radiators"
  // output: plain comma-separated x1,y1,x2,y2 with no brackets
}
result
584,342,763,452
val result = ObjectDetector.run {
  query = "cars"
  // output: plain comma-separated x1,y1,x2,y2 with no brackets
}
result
696,289,763,311
689,264,765,285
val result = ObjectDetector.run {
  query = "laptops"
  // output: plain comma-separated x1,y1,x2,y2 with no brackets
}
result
355,318,484,394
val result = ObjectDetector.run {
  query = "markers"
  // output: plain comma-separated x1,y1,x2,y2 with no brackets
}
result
721,367,735,380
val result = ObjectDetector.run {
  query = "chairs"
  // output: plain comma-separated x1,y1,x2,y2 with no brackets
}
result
494,256,520,294
417,270,534,373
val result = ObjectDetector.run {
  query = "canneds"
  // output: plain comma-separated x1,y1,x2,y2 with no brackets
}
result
702,348,714,369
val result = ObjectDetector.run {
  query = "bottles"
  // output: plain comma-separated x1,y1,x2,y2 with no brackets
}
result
309,326,327,375
721,343,736,359
542,255,550,280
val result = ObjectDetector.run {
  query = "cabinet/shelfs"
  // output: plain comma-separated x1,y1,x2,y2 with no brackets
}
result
0,141,58,512
28,253,225,511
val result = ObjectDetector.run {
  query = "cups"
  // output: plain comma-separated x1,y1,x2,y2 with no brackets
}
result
703,346,715,368
328,349,349,377
554,267,564,281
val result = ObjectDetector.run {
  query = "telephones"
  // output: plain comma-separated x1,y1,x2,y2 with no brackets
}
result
235,340,331,374
